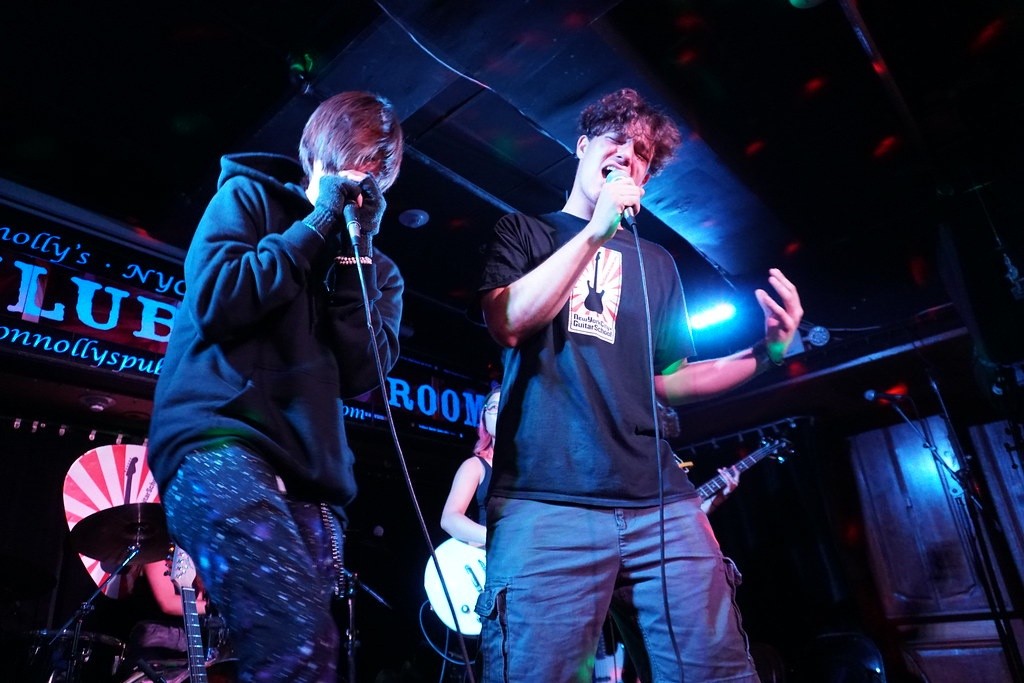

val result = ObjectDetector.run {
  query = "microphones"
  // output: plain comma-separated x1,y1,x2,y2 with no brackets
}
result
606,170,637,227
866,390,906,401
344,200,361,247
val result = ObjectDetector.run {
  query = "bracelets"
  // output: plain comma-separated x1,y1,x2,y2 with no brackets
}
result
753,337,785,373
711,494,721,507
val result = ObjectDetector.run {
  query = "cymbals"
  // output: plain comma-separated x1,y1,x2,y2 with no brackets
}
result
72,503,175,564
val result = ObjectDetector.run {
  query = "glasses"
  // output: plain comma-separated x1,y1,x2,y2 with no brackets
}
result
483,401,499,415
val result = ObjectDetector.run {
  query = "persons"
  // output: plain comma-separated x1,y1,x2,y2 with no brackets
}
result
147,92,410,683
439,385,740,683
130,512,207,683
478,92,809,683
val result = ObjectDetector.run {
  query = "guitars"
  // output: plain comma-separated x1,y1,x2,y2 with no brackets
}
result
166,540,210,683
694,436,797,502
423,534,484,638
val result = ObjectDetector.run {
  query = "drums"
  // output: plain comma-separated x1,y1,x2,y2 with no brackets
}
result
26,627,129,683
